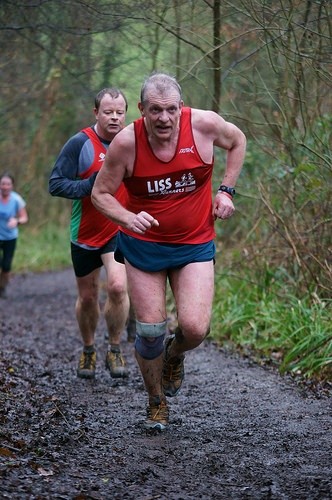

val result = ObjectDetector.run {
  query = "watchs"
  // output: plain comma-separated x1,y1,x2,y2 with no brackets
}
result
219,185,235,196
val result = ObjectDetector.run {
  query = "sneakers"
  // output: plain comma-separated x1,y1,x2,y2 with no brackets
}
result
161,335,185,397
105,345,130,378
148,395,170,428
78,349,98,379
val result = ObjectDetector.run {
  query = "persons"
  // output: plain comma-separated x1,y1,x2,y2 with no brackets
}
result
0,174,29,299
48,88,130,377
91,73,247,435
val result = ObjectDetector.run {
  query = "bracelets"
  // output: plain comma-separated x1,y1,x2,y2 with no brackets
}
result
218,190,233,200
